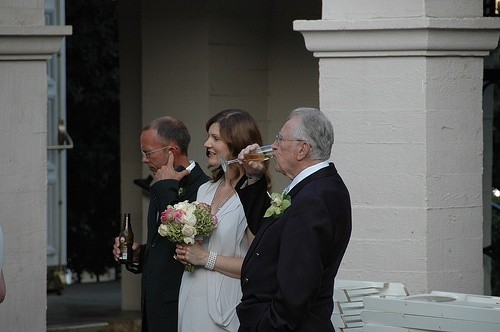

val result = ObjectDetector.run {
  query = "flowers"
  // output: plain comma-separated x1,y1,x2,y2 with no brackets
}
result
157,200,218,274
263,189,291,218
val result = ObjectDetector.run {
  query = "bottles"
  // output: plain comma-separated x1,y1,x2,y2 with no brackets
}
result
119,213,133,263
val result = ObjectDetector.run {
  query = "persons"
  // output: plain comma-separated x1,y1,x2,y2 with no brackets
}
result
111,115,213,332
0,226,6,303
234,107,353,332
174,109,273,332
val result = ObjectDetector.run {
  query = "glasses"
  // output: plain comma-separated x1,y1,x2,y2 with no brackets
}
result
141,147,168,158
274,134,306,146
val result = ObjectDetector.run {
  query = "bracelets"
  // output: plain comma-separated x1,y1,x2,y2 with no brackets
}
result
246,173,260,180
204,250,218,270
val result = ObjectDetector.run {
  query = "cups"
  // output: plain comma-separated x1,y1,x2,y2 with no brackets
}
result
220,145,274,173
